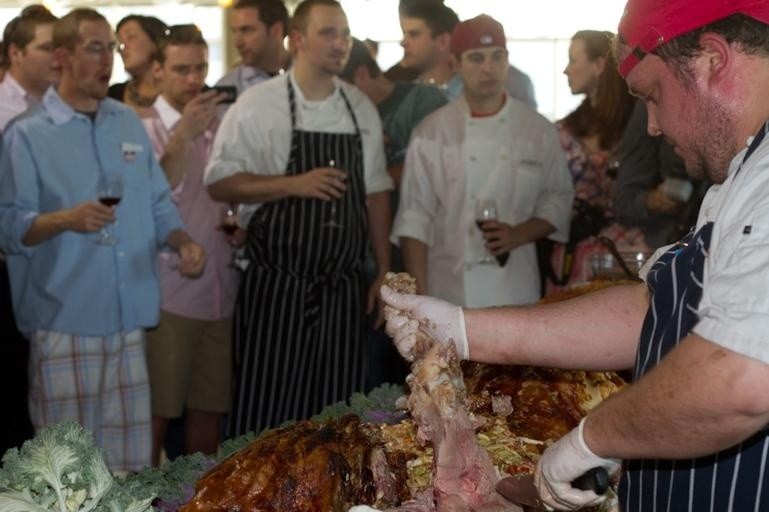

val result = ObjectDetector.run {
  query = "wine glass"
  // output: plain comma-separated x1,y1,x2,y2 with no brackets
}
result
221,209,239,256
605,158,622,209
323,159,348,230
474,197,499,265
97,171,124,246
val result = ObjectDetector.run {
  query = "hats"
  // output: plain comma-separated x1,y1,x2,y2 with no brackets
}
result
613,0,769,81
450,14,507,55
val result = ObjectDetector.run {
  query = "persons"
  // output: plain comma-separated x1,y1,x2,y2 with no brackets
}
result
0,1,717,466
2,9,206,471
389,16,574,309
205,0,396,449
380,0,767,512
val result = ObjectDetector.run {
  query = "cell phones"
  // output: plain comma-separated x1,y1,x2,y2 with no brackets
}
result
202,86,237,104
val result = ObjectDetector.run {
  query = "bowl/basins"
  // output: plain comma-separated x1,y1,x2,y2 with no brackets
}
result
588,249,654,282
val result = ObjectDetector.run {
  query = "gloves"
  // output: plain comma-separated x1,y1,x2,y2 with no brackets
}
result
531,414,623,512
378,283,472,372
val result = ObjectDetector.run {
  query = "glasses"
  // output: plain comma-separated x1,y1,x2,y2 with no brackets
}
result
71,43,127,60
